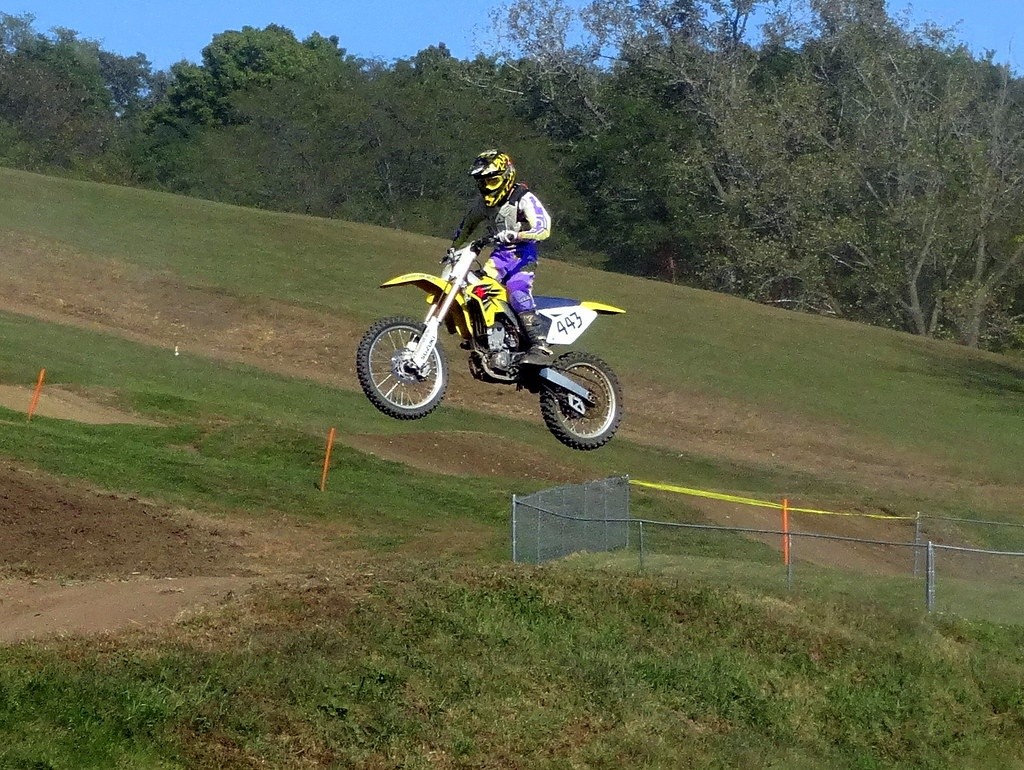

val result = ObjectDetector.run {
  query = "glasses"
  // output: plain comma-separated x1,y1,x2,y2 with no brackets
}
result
474,176,503,190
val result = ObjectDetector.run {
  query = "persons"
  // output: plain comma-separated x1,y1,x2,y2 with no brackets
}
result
447,149,554,358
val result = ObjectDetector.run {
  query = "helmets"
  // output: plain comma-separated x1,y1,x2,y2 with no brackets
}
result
468,150,516,207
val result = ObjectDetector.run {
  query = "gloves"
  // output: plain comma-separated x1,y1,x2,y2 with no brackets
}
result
493,230,518,245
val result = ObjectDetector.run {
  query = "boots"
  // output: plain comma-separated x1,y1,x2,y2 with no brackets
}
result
518,309,553,366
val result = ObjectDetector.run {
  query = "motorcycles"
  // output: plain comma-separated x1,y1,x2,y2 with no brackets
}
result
356,233,624,451
